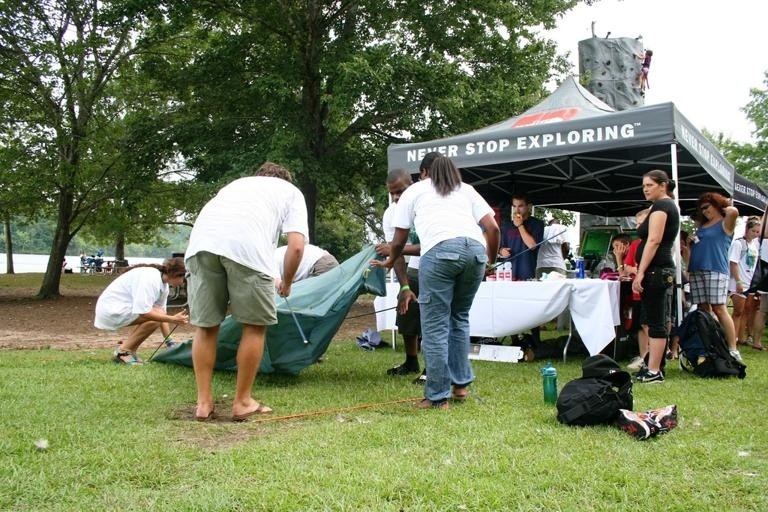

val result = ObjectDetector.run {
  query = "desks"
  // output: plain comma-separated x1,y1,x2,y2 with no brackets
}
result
370,275,623,366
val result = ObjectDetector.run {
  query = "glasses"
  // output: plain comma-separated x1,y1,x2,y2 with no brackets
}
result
701,204,711,210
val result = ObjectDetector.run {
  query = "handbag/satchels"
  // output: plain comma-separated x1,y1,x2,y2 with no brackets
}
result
556,370,632,427
677,309,746,380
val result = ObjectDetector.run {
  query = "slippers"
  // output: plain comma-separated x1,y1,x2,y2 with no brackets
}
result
232,402,272,420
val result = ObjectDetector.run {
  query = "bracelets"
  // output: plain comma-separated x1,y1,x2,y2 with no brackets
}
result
486,263,496,270
517,223,524,227
164,336,174,344
401,285,410,294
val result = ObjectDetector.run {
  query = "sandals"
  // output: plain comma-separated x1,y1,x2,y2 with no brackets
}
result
112,348,145,366
418,386,470,408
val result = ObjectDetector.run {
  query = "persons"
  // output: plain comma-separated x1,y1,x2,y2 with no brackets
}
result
536,220,571,330
500,195,545,349
612,170,680,383
374,169,429,385
184,163,310,422
62,250,112,273
681,193,767,359
93,257,190,366
634,50,653,90
369,153,500,409
275,244,339,284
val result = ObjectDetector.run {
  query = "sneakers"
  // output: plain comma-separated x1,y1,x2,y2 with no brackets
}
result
414,368,427,383
616,404,677,441
628,356,665,383
386,364,420,374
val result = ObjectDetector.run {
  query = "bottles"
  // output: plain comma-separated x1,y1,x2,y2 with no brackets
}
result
486,262,512,280
541,361,558,404
575,256,584,279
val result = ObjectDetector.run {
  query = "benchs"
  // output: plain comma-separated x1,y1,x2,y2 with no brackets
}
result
79,258,129,276
